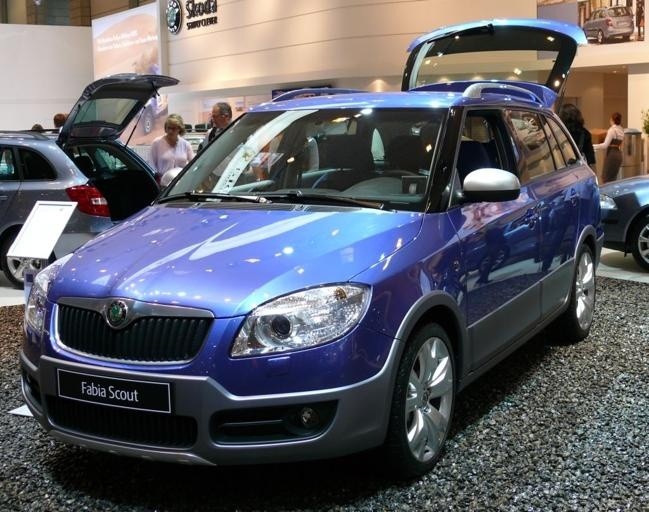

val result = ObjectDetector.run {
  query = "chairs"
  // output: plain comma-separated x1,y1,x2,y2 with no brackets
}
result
318,137,488,192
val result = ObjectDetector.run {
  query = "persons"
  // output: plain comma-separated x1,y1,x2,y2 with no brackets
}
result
31,124,43,131
151,113,195,181
268,133,319,178
636,2,643,36
593,112,625,184
518,113,549,178
197,102,264,192
559,104,596,167
53,113,66,128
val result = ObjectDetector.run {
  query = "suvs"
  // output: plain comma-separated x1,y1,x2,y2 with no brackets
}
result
18,19,607,479
583,5,634,43
0,73,180,292
597,177,648,272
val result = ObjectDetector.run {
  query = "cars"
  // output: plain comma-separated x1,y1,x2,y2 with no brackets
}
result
141,63,169,134
